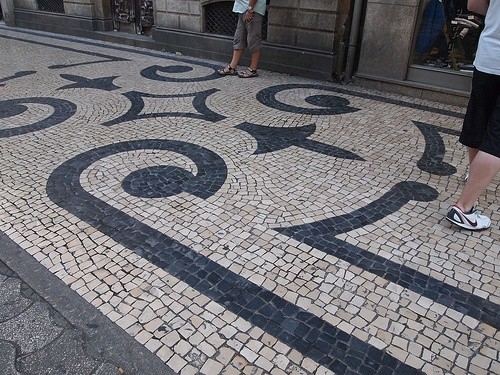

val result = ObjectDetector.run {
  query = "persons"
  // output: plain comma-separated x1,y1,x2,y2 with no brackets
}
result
216,0,267,78
444,0,500,231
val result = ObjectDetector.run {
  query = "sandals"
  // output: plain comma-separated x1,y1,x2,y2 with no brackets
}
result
217,64,259,78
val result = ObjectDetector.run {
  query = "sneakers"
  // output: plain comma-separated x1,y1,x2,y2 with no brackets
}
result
464,173,469,183
445,206,491,231
